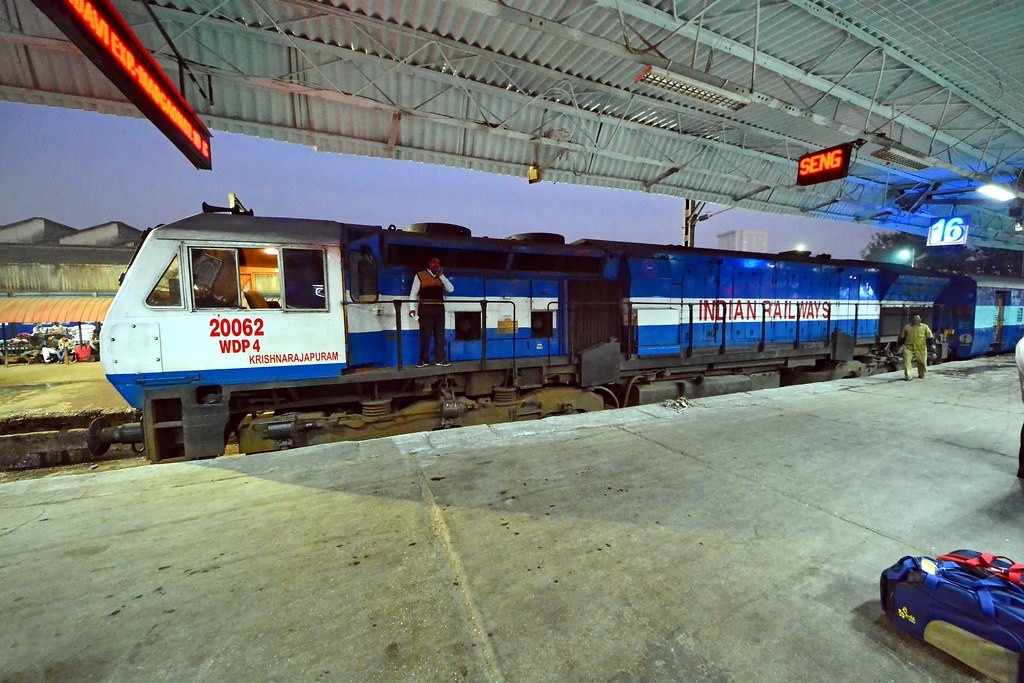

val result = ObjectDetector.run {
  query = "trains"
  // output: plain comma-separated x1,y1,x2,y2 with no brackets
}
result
102,193,1024,464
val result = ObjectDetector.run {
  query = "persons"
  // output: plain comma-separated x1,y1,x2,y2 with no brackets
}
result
408,255,454,368
896,312,935,381
1015,337,1024,478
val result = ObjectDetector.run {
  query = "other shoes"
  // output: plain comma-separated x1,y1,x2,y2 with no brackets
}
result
435,361,450,366
416,363,429,368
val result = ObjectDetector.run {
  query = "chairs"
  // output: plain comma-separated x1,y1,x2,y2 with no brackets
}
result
245,290,268,307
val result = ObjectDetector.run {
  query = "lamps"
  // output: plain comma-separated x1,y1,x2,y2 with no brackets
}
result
974,183,1018,201
635,66,753,115
528,169,543,185
870,146,934,171
